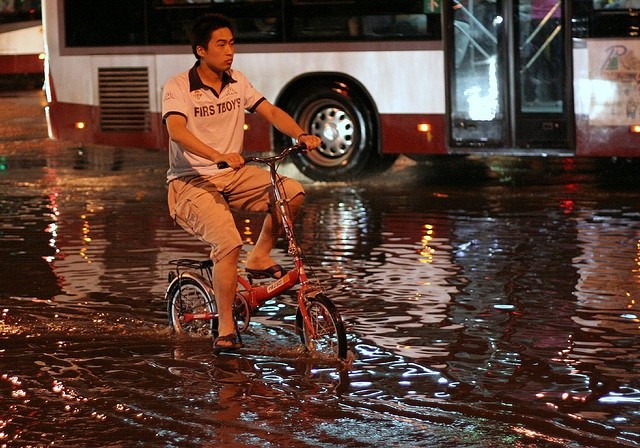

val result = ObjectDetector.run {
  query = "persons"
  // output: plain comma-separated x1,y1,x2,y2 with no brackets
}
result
162,14,323,352
524,0,562,107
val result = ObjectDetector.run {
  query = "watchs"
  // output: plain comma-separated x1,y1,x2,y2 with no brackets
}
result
296,133,308,142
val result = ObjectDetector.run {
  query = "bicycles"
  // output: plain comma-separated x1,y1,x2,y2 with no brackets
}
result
164,140,348,361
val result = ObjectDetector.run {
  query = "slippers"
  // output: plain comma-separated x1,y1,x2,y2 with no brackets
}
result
213,334,237,351
245,264,287,284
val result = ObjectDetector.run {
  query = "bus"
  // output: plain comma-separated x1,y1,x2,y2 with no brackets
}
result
40,0,640,183
